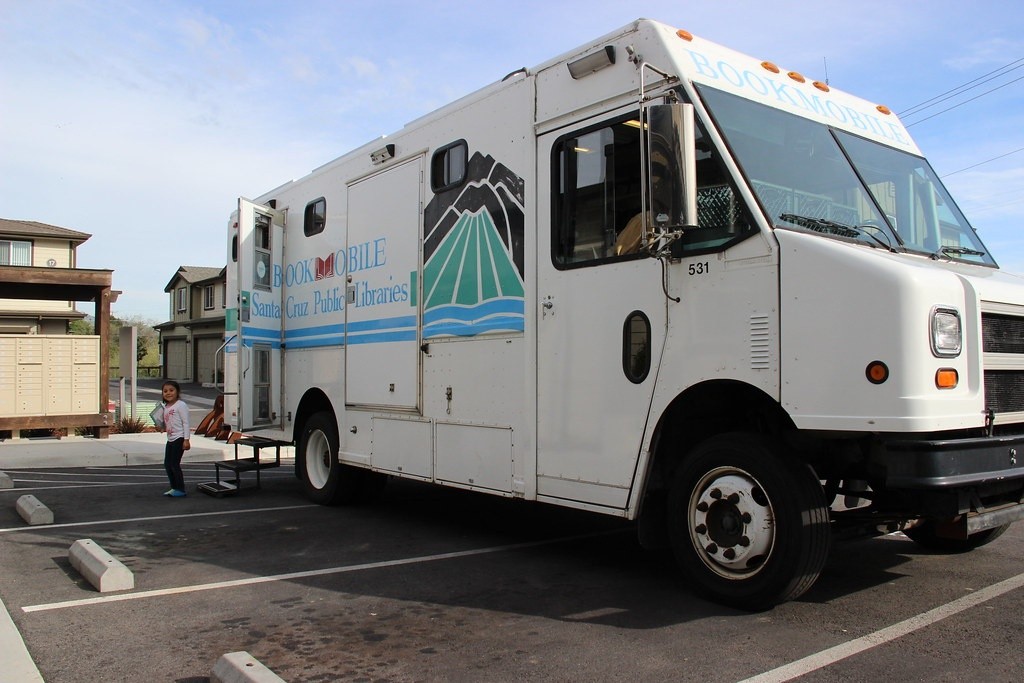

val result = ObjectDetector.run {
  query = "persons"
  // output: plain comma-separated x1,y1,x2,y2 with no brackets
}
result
153,381,191,496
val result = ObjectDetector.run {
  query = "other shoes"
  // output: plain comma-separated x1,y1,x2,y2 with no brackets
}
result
164,490,187,496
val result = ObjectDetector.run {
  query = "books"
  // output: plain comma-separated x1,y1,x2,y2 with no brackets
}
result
150,403,165,429
